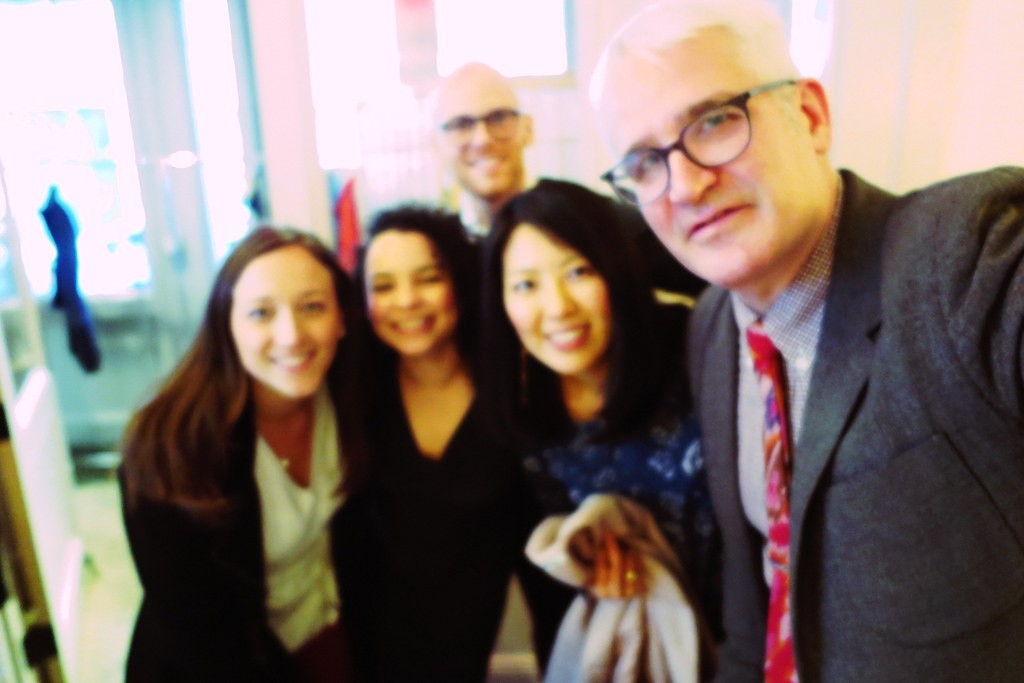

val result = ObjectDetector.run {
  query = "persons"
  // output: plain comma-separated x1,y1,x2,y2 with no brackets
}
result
118,229,403,683
352,208,516,683
480,178,725,683
431,61,580,683
587,0,1024,683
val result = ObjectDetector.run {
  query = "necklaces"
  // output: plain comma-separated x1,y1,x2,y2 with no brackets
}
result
279,411,308,467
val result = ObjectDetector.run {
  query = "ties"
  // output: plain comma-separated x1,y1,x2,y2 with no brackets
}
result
746,316,800,683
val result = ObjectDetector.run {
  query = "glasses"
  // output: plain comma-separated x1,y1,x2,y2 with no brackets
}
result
439,109,521,154
601,78,795,206
